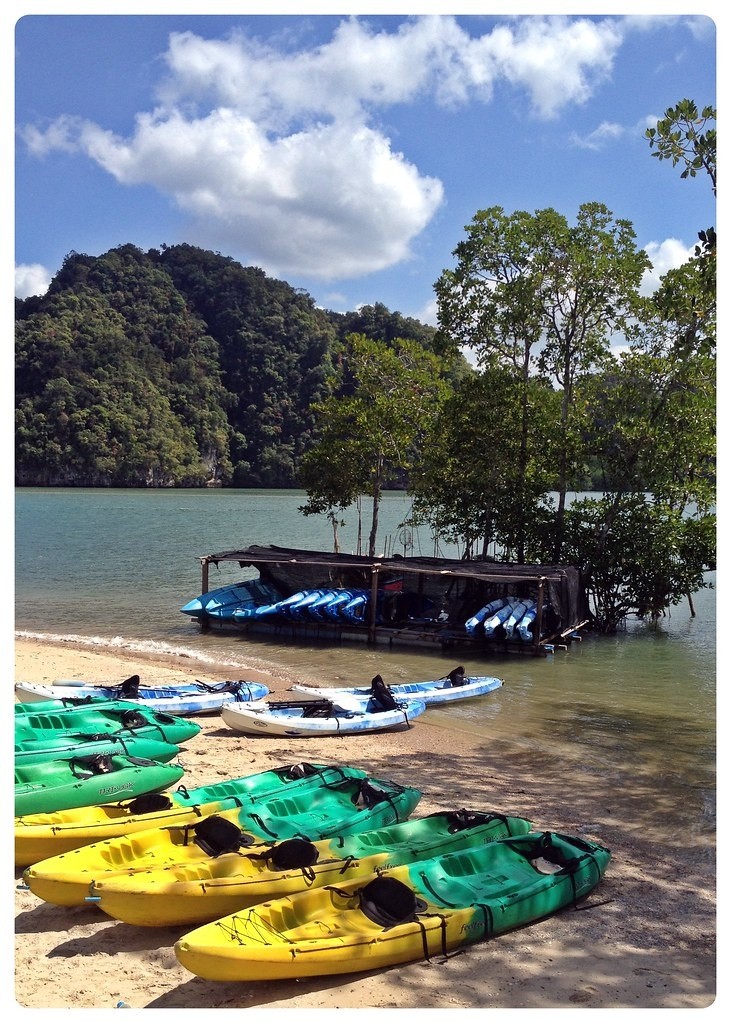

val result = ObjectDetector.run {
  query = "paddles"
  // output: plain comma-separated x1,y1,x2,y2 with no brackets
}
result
235,699,333,710
53,679,187,693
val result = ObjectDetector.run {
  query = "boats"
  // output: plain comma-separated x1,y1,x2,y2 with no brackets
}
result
84,806,532,927
15,696,200,764
179,577,553,642
16,674,269,715
175,831,611,982
21,776,421,906
14,752,183,818
291,667,504,705
220,682,426,736
15,760,366,865
